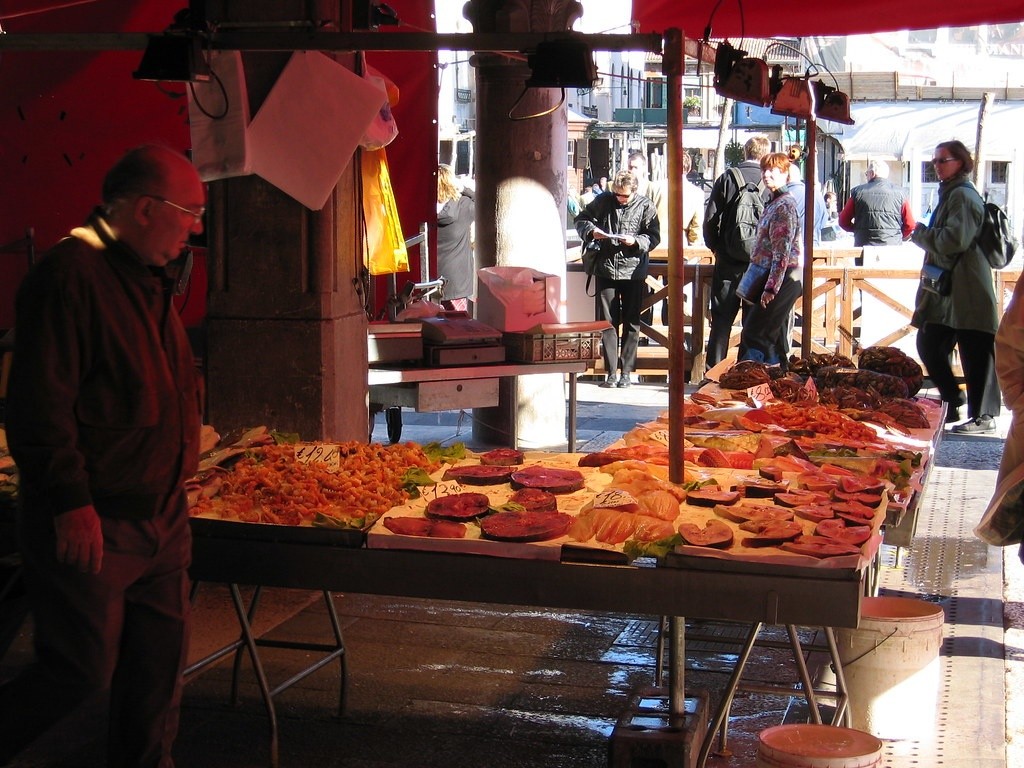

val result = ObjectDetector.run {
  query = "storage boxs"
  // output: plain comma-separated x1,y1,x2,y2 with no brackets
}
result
367,321,424,363
507,332,604,362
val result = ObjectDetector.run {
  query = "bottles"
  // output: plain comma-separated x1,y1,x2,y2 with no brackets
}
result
587,238,600,251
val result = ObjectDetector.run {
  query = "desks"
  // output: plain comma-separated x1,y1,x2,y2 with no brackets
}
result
0,365,950,768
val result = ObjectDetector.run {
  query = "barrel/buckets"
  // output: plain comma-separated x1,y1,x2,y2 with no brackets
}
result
831,595,944,738
756,723,882,768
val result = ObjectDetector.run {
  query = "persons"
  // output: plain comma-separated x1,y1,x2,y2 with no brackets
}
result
977,270,1024,569
702,137,778,380
437,163,475,311
574,170,661,388
735,153,803,363
822,192,837,241
838,160,915,303
910,140,1002,435
692,152,713,192
1,145,206,768
566,177,608,229
786,163,829,247
626,151,705,346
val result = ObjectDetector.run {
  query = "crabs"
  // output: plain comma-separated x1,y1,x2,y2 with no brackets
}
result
717,359,931,436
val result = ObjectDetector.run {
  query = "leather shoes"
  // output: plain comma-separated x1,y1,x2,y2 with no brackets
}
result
606,374,617,387
946,405,961,422
952,418,996,434
618,372,630,387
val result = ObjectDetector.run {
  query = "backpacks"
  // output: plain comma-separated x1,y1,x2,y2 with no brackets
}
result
965,185,1019,270
727,167,766,265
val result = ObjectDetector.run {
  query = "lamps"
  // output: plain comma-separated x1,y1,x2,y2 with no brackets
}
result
133,31,213,85
773,74,815,125
523,42,600,92
707,40,772,111
816,86,859,127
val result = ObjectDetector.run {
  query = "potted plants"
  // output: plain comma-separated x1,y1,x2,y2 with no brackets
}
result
684,96,700,116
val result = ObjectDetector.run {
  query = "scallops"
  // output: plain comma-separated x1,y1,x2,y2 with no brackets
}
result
788,346,924,401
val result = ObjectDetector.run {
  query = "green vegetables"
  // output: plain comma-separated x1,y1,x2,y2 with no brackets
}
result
312,511,367,529
398,440,466,499
621,479,717,565
201,425,301,474
810,447,923,490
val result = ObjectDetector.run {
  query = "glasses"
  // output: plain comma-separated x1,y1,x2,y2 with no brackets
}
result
932,157,958,164
613,191,634,197
141,194,204,223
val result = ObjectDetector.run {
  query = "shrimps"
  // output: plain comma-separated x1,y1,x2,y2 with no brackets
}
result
763,402,880,448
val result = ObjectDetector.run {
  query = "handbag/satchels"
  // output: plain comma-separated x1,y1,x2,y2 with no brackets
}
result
920,265,951,298
581,191,611,275
735,262,771,306
